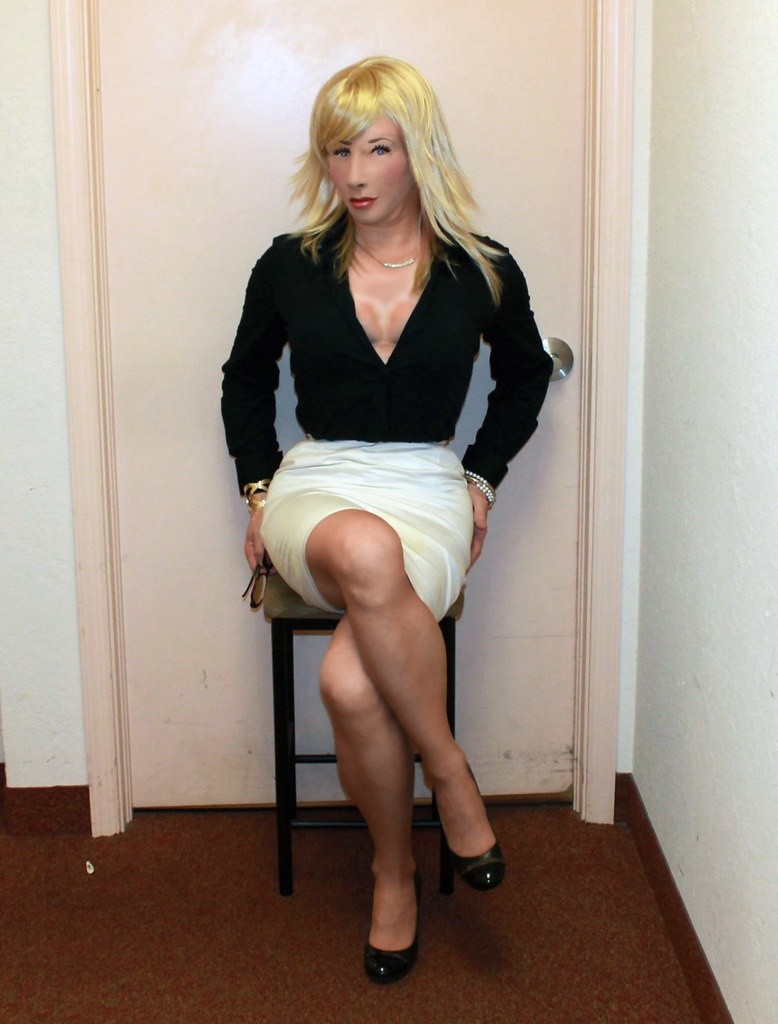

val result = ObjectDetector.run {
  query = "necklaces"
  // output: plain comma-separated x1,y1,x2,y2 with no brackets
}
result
355,213,423,268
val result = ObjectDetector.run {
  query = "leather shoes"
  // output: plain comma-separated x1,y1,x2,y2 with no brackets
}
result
431,763,505,892
364,868,422,984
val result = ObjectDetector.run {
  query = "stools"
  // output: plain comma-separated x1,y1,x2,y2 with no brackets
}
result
263,567,465,898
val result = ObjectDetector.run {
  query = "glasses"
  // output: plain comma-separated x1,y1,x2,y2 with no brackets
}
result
242,547,273,608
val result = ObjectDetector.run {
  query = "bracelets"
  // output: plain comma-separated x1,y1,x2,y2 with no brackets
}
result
463,471,496,505
244,479,271,513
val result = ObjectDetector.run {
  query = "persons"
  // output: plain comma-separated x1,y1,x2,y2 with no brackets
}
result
220,56,554,986
